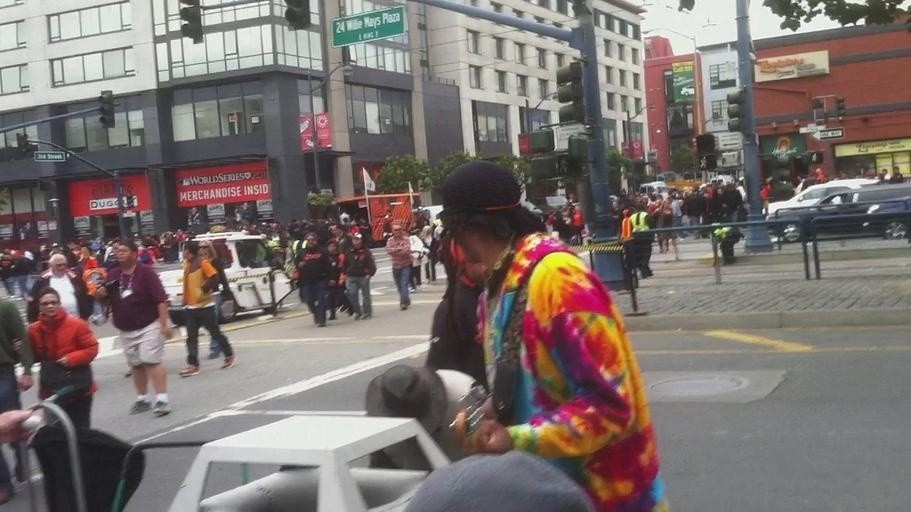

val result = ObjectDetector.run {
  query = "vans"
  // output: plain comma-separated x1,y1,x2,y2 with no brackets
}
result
710,175,735,194
414,205,445,228
639,182,668,202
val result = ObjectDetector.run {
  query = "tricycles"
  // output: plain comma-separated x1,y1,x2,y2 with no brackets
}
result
156,231,297,325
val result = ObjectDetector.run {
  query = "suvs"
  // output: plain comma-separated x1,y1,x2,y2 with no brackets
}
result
762,178,886,243
534,196,569,223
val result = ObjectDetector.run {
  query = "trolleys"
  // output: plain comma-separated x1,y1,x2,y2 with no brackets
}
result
20,379,205,511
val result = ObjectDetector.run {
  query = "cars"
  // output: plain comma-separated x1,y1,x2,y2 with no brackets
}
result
767,182,911,241
681,85,695,97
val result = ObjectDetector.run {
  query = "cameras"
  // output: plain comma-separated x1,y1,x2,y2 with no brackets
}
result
96,277,119,289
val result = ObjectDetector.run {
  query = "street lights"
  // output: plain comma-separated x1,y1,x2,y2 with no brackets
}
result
29,175,62,276
308,59,356,194
525,92,558,133
700,115,724,133
640,26,707,185
627,103,657,160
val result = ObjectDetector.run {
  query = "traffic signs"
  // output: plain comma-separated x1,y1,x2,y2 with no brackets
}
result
813,128,844,141
34,151,67,163
330,6,407,49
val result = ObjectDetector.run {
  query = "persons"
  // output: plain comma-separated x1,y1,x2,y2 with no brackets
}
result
0,201,377,416
0,286,98,505
877,166,903,181
610,169,825,280
364,162,668,511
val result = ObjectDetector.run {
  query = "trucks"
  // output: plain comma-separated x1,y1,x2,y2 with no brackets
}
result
337,192,422,246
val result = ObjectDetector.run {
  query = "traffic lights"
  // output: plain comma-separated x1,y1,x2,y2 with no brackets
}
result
696,134,718,171
557,153,570,173
127,194,136,210
728,90,747,133
811,150,823,163
284,0,311,30
568,135,586,163
179,0,204,45
16,132,30,158
99,90,116,129
557,62,586,124
836,96,846,117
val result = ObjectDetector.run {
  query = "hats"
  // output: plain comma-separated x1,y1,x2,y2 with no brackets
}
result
440,159,521,211
405,449,593,511
366,363,447,434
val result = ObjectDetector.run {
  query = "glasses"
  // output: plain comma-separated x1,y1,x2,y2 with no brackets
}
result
200,245,208,249
57,263,68,267
40,300,58,306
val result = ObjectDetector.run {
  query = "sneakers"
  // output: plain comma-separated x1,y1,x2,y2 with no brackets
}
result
179,365,201,377
221,353,237,369
128,400,152,414
152,400,173,414
